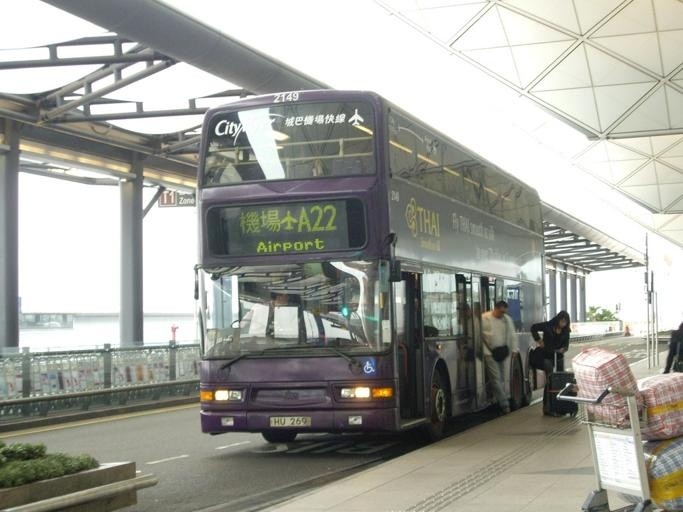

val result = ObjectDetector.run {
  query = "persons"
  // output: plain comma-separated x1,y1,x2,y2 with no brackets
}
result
662,321,683,373
480,300,520,413
530,310,571,383
240,287,290,336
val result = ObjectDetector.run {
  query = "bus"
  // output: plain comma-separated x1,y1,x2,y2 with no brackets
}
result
188,89,546,443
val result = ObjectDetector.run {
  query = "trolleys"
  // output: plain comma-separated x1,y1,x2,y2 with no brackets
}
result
554,384,683,512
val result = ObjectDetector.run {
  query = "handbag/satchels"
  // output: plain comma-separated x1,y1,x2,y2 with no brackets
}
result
637,373,683,440
643,436,683,512
572,347,644,425
492,345,509,362
529,347,555,370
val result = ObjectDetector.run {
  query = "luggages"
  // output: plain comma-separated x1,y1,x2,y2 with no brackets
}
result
543,350,578,417
